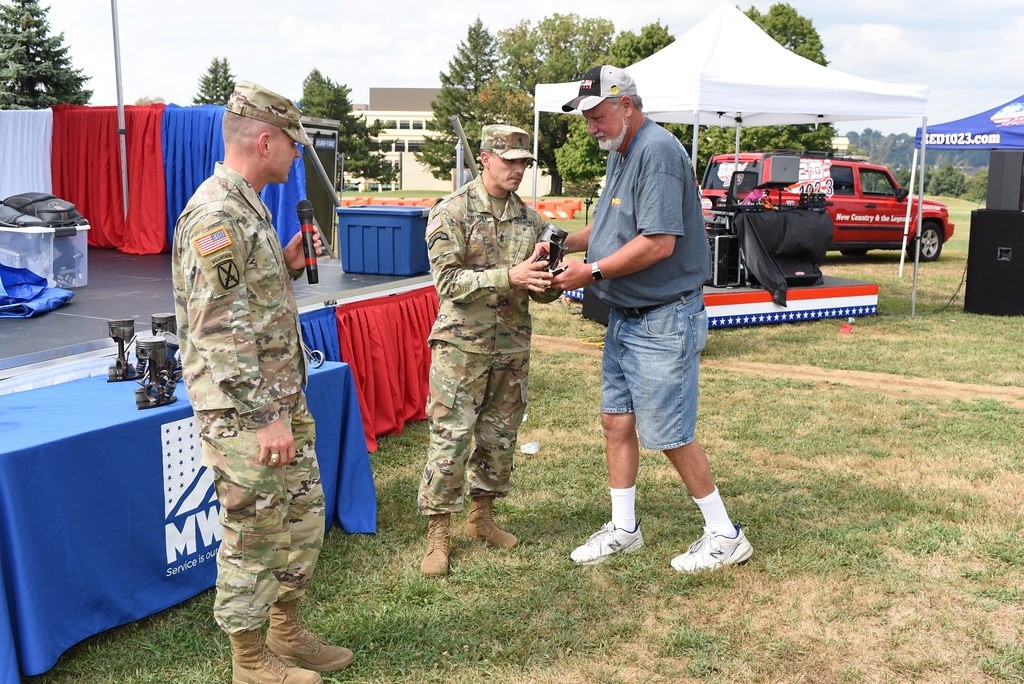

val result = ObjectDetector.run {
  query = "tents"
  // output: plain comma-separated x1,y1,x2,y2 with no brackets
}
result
898,95,1024,278
531,0,928,318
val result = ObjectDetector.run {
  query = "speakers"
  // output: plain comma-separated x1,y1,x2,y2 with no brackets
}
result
758,156,800,188
772,254,819,288
987,150,1024,211
964,209,1024,317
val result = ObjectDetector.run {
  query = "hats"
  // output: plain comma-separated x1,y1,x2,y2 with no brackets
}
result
224,81,313,145
480,124,536,161
562,64,637,112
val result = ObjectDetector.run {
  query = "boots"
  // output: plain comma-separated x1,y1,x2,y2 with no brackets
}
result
265,598,355,672
420,513,452,577
228,627,324,684
463,496,518,548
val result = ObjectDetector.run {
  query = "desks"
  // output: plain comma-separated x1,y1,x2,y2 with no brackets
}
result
0,348,375,684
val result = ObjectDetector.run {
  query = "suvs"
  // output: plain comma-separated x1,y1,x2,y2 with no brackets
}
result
699,149,955,262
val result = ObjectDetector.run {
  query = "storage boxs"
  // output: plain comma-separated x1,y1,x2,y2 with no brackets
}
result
336,205,433,276
0,225,91,288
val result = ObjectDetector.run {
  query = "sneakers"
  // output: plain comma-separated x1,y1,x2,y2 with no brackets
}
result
670,525,754,574
568,517,648,565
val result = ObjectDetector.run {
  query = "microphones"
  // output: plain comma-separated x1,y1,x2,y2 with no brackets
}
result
297,200,319,284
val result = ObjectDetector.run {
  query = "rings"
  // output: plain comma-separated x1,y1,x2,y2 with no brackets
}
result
271,453,280,463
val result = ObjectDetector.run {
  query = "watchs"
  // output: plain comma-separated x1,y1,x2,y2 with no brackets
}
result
591,261,604,280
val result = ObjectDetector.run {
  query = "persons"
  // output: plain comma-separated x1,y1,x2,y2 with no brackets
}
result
535,64,753,575
172,78,353,684
417,123,554,577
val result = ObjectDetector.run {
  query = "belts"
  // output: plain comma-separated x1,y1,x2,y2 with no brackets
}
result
616,291,694,318
